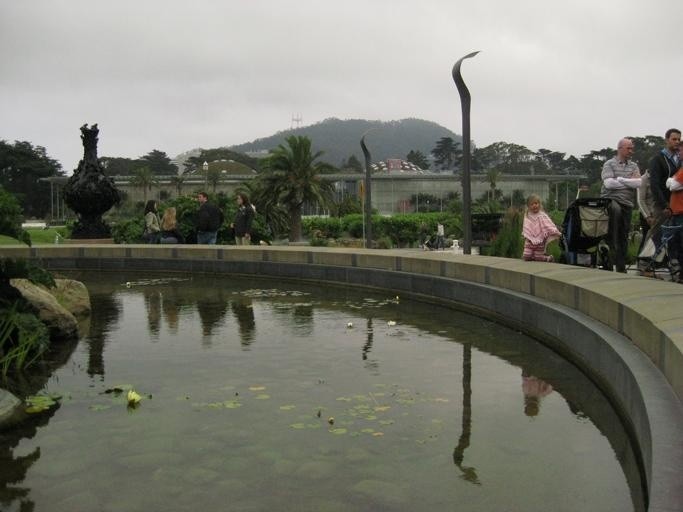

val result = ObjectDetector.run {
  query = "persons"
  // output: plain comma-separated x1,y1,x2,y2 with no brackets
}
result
194,190,222,244
143,287,162,339
664,140,682,285
635,155,657,273
160,286,182,334
159,206,185,243
229,191,255,245
144,198,161,243
647,127,681,266
519,367,551,417
522,192,561,263
600,136,643,274
195,294,218,338
230,298,256,350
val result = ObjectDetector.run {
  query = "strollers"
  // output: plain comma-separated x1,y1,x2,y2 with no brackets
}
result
623,208,683,283
556,198,612,266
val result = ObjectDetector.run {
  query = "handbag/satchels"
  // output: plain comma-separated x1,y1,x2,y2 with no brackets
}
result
143,230,149,240
